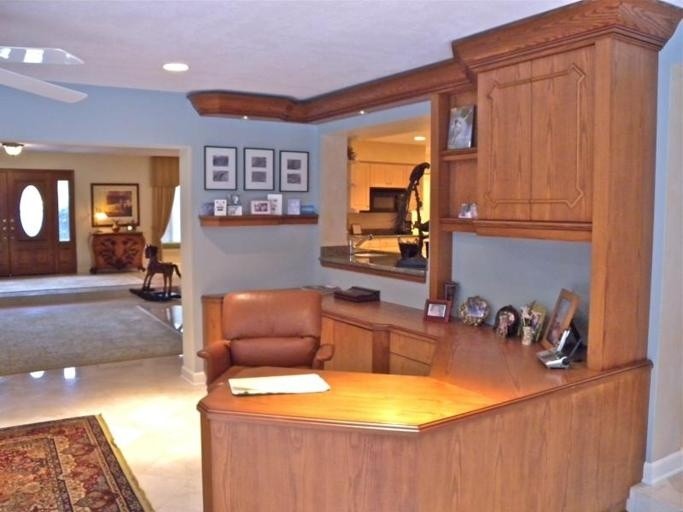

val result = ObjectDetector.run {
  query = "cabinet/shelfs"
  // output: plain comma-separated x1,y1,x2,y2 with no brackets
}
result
431,0,683,370
319,310,390,373
389,325,438,377
201,295,222,348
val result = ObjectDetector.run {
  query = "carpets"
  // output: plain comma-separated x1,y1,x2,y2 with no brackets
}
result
0,303,183,376
0,414,156,512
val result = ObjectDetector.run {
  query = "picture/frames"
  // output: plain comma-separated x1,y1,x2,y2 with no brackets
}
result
424,299,451,323
540,289,579,350
204,145,238,190
250,200,271,215
243,147,276,191
279,150,309,192
91,182,140,227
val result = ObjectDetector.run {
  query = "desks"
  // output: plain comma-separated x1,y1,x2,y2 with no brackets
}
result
89,232,146,275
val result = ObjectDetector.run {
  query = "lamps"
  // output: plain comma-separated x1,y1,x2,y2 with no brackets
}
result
94,212,108,233
3,143,24,157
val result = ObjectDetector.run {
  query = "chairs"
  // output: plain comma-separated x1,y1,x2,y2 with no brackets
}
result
196,290,335,393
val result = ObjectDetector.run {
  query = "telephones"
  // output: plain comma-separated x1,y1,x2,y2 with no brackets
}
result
536,326,584,370
